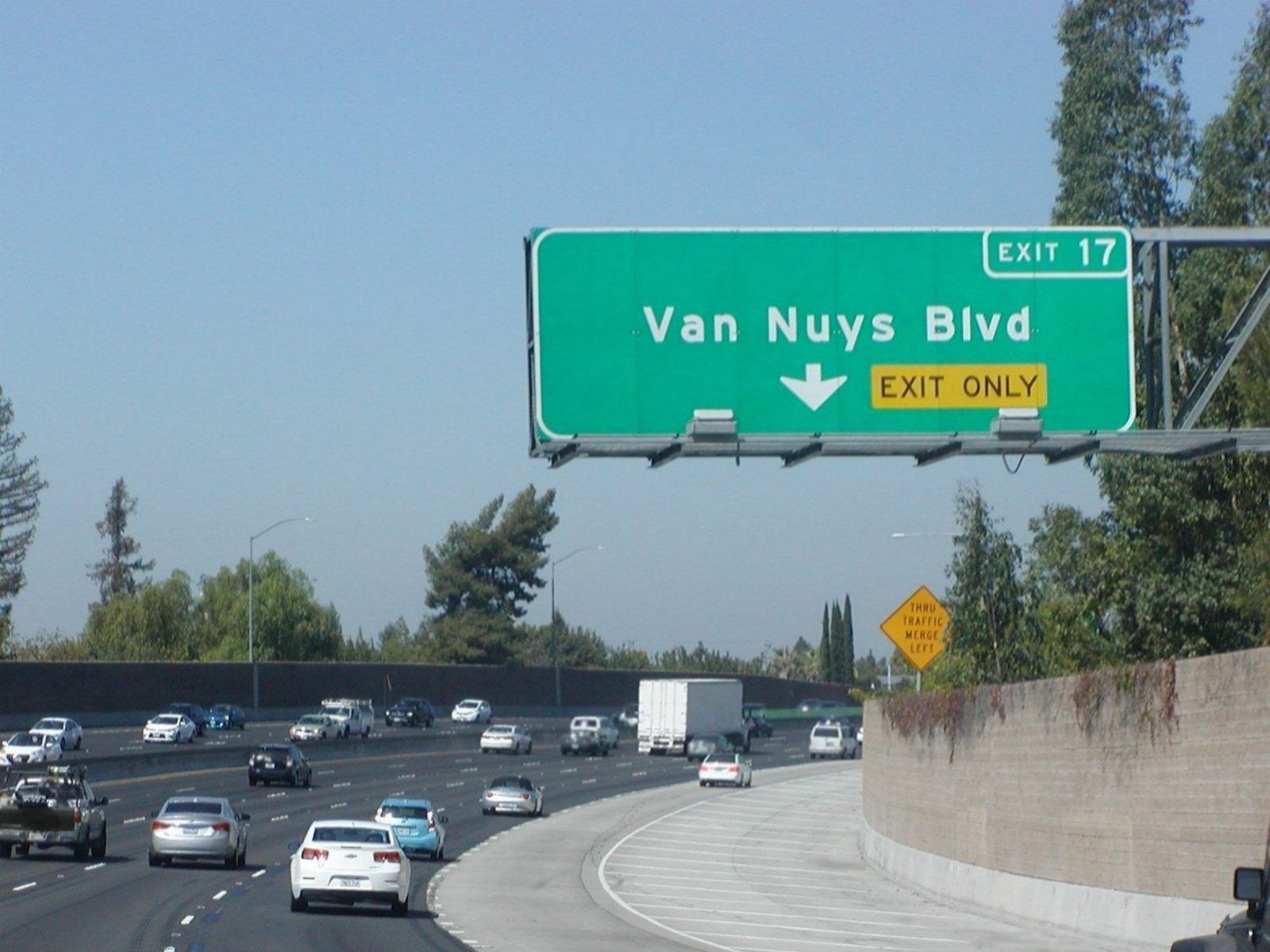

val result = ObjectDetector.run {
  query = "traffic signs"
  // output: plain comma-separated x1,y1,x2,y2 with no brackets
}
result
527,222,1140,447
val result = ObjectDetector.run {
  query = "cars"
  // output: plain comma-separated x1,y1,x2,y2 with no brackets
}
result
287,819,416,918
149,795,253,869
208,705,248,731
290,714,346,743
559,730,610,756
480,724,532,754
29,717,83,750
143,713,198,743
1,733,63,766
160,701,206,736
796,698,849,708
375,796,450,858
686,731,735,761
450,698,492,725
248,743,312,786
744,715,773,738
699,753,752,788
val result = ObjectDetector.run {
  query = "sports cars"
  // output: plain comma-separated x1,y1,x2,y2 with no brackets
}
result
482,775,546,815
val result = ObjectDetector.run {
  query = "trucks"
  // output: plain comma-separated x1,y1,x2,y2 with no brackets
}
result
638,677,751,756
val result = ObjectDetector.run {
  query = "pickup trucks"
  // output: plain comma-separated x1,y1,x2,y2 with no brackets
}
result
319,704,374,738
0,767,110,863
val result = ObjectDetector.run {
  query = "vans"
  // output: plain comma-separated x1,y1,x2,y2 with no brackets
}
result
808,718,858,759
570,716,620,747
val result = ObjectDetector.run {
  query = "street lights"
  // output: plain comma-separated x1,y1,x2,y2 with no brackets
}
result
551,545,602,704
247,517,310,711
892,530,972,584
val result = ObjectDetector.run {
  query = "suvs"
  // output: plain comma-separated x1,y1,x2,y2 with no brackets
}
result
385,697,435,726
1169,825,1270,951
